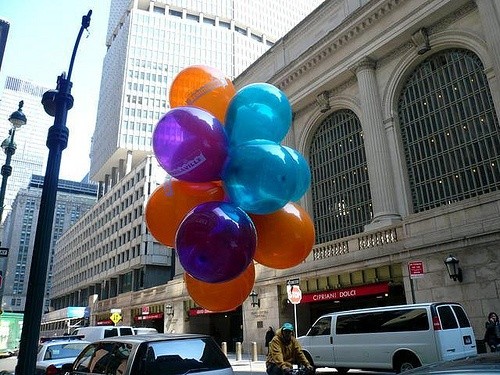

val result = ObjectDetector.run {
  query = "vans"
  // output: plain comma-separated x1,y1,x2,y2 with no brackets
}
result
295,302,479,374
69,326,159,343
62,332,233,374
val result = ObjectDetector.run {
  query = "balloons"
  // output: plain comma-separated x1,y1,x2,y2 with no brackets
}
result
146,65,315,312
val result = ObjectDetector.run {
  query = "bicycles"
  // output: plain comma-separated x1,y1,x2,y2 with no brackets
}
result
284,366,311,375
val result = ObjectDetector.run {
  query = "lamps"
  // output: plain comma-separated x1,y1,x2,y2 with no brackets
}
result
315,91,330,114
443,255,463,284
165,303,174,318
411,29,431,56
248,290,260,307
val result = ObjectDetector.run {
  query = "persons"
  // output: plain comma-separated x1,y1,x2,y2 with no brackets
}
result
266,322,313,375
265,326,275,357
484,312,500,352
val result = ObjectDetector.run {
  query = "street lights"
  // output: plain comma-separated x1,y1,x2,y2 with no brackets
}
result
0,100,28,224
67,318,88,334
15,71,75,375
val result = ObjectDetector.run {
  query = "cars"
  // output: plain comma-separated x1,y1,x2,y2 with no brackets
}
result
36,334,92,375
403,352,500,374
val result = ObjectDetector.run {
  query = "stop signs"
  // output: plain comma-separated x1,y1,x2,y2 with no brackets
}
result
287,286,303,305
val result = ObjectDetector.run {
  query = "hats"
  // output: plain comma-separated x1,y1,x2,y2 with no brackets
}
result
282,323,294,331
